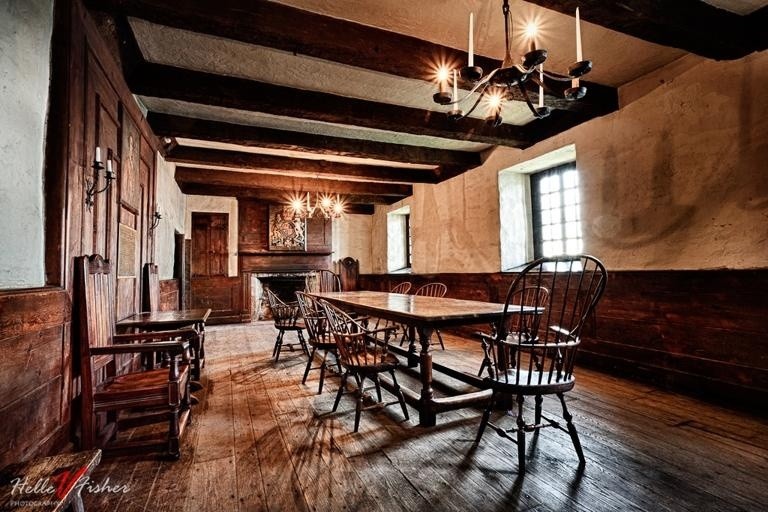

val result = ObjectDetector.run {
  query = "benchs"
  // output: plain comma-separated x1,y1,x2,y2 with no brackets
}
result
1,446,102,512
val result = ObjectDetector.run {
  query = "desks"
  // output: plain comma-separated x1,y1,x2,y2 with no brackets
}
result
114,308,216,404
309,290,545,440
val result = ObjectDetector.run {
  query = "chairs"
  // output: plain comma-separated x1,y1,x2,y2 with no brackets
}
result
307,268,342,294
399,282,448,351
474,252,607,477
492,286,551,372
373,281,413,340
293,288,342,395
74,253,204,462
319,297,411,433
265,287,312,362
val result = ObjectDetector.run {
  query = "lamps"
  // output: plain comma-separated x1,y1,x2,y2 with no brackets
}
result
429,1,596,131
86,144,116,205
149,203,162,230
